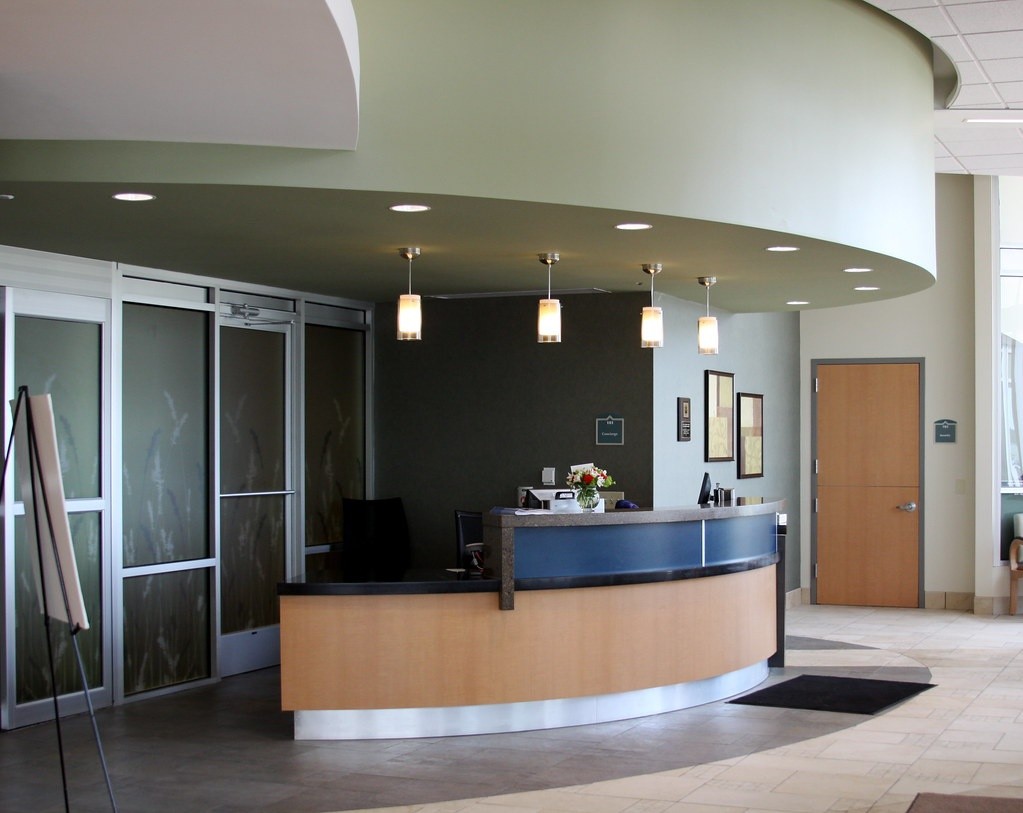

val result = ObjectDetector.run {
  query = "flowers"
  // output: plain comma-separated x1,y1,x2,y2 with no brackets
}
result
565,461,618,512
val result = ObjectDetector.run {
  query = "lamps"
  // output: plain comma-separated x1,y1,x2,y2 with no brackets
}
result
695,275,721,358
394,243,427,342
639,262,666,349
535,250,564,346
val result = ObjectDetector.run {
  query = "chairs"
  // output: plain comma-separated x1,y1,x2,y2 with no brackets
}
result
1009,535,1023,616
455,509,484,576
328,491,412,582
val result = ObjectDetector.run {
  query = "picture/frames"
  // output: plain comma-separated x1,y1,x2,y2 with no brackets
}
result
703,369,736,463
735,391,766,480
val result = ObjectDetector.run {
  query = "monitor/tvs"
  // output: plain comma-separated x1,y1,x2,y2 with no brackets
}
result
524,488,605,515
697,472,711,504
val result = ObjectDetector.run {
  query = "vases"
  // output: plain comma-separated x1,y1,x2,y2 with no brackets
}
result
577,486,603,508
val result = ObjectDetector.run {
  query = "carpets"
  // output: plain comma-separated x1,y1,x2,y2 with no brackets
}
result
726,673,939,716
906,791,1023,813
